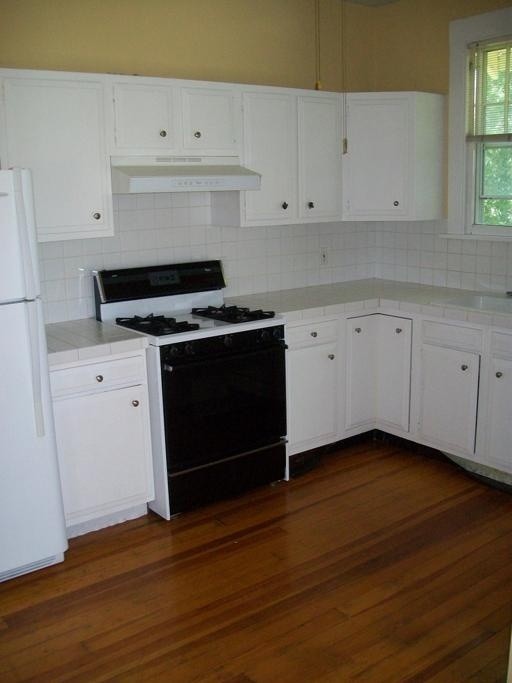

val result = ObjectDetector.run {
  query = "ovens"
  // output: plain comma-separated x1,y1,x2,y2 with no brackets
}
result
148,334,293,522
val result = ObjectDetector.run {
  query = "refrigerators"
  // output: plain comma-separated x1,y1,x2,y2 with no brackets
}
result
1,165,70,586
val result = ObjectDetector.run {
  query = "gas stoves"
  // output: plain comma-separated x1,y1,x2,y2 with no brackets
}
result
98,288,276,338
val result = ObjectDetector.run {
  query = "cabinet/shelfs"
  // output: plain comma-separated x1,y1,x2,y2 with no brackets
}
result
343,302,416,441
285,315,343,454
1,67,115,242
345,89,443,222
47,348,155,538
210,81,343,227
416,316,511,472
108,73,238,160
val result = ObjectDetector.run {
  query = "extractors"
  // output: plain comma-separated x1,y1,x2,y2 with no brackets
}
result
110,153,261,193
